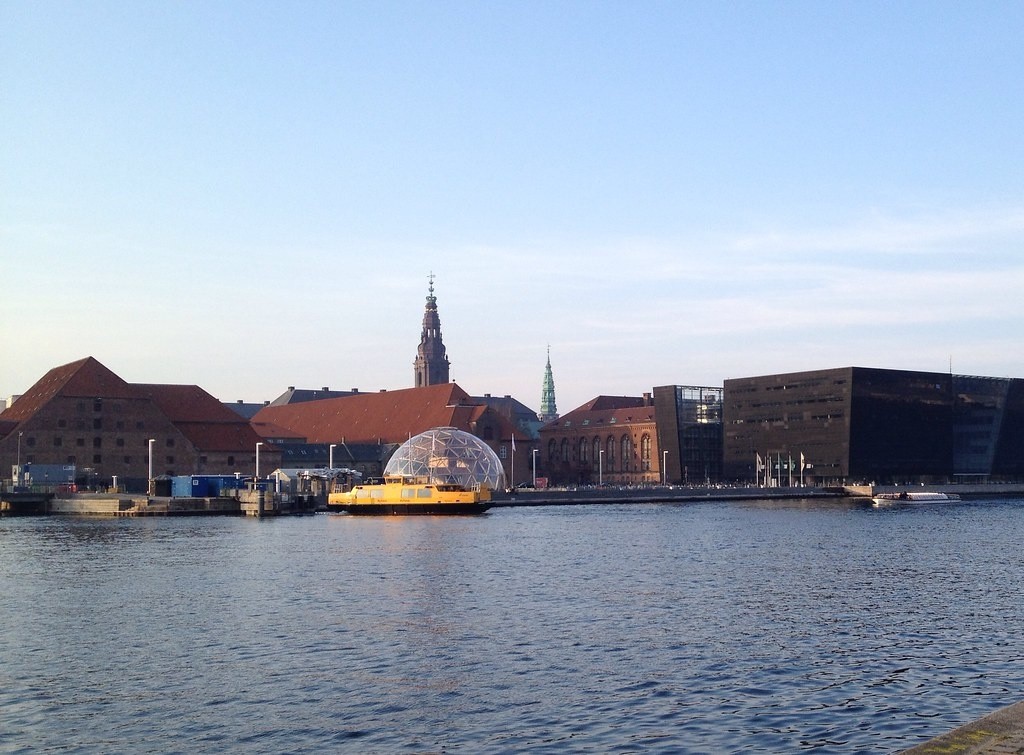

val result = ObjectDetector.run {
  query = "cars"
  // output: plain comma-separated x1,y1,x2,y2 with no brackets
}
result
655,482,674,488
637,482,651,489
518,482,534,488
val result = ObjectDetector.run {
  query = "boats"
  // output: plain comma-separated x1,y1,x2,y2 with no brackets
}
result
871,490,962,508
327,475,496,516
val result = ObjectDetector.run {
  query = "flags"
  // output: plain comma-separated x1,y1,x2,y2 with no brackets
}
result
779,454,783,471
801,453,804,471
512,434,517,451
757,454,762,472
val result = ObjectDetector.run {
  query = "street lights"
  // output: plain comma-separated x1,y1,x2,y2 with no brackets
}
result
533,449,539,486
330,444,337,469
600,450,604,485
18,431,23,472
149,439,156,492
664,451,669,485
256,442,263,476
